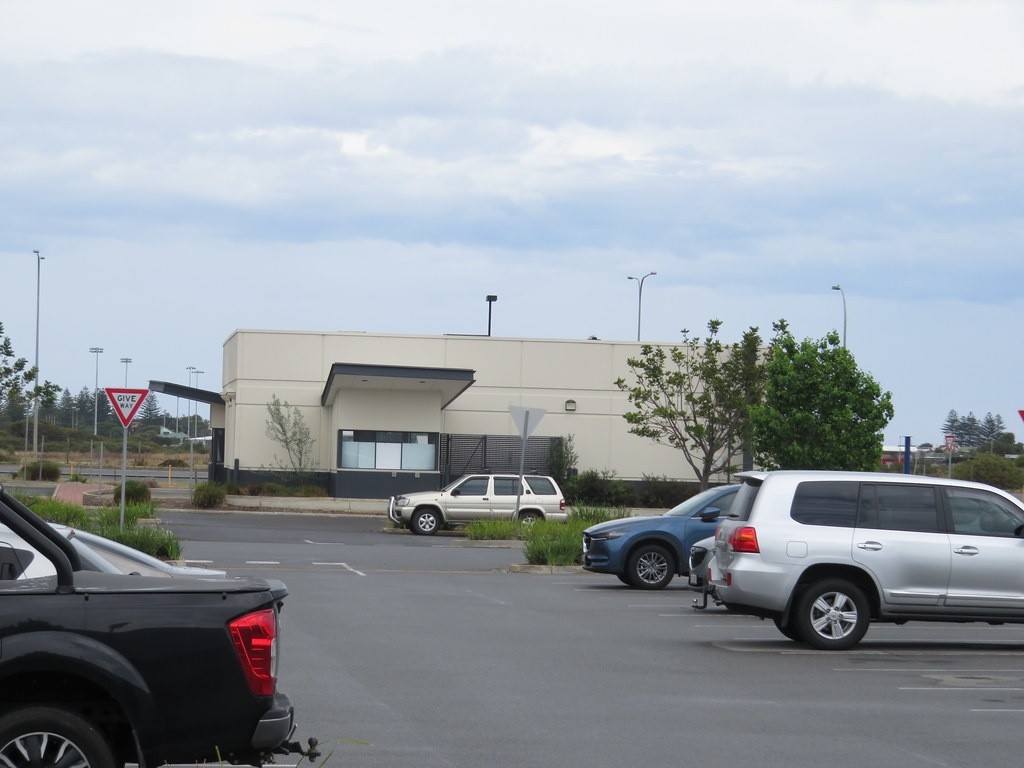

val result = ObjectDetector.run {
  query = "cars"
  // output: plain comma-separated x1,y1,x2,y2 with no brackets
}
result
0,486,326,768
688,537,721,604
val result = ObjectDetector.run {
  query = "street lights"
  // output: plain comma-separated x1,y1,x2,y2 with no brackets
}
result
832,285,847,350
627,271,657,342
32,250,45,462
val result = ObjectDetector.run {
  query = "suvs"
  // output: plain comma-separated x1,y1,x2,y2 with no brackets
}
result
387,473,568,541
581,484,744,590
713,469,1024,651
